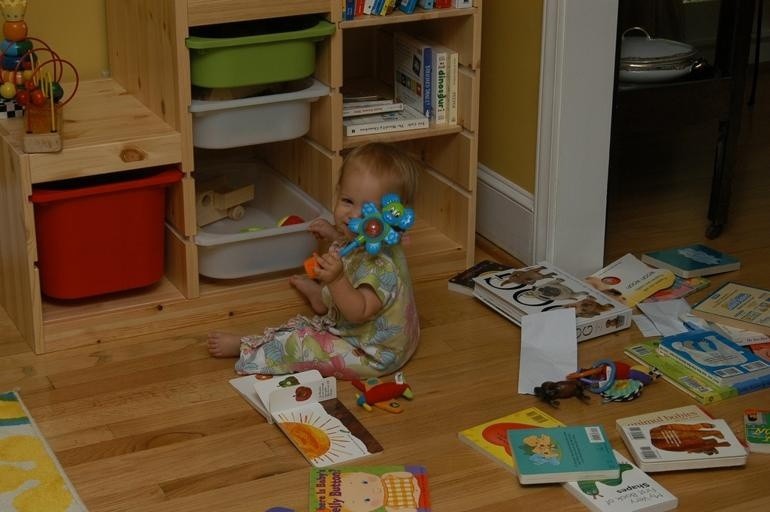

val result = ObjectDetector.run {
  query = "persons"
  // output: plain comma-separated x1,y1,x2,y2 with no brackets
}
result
206,141,420,380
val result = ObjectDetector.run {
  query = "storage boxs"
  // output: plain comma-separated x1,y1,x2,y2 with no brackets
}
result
30,168,182,300
184,21,336,282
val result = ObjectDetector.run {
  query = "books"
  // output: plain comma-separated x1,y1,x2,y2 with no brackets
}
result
642,242,743,279
308,461,432,511
616,403,751,458
342,0,474,23
342,33,460,140
693,282,769,336
644,278,712,303
562,449,679,511
589,253,677,308
658,330,770,385
448,261,514,299
625,337,770,403
225,372,383,469
457,406,567,475
507,424,621,485
471,262,633,344
621,418,748,473
743,407,770,455
680,313,769,348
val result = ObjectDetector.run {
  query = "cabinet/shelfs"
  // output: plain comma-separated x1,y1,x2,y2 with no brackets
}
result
0,0,481,358
